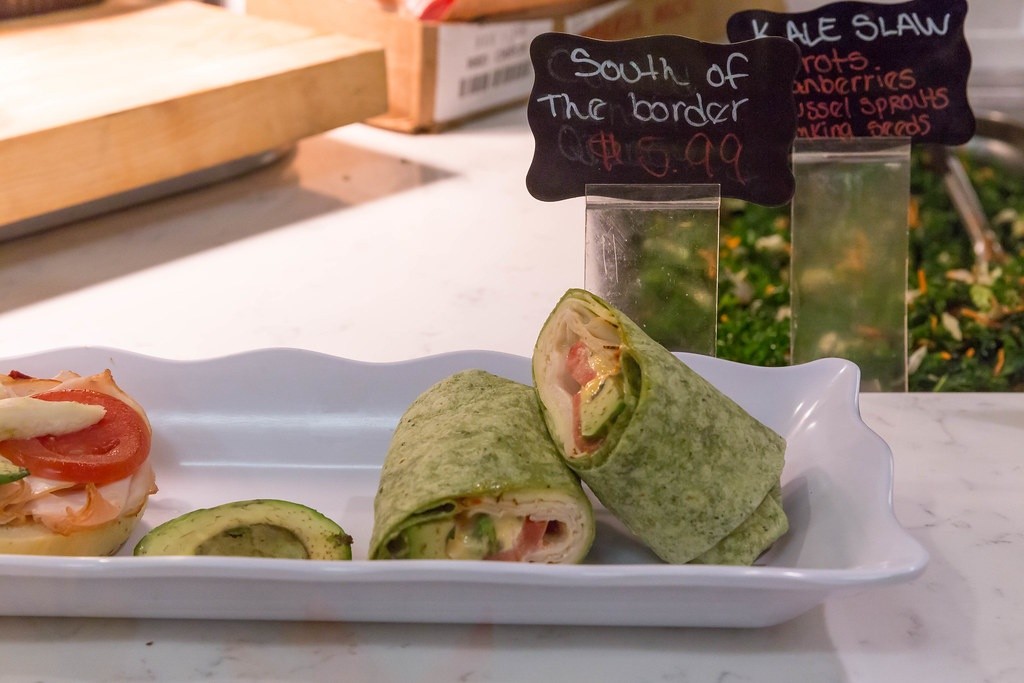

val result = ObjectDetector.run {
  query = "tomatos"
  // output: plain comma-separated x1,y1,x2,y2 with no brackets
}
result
0,388,150,483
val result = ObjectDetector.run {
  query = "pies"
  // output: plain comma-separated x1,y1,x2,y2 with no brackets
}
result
126,290,790,578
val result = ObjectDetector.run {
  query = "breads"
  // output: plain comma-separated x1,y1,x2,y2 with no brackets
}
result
0,496,148,557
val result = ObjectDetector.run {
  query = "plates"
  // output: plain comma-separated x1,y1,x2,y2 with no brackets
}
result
0,348,930,628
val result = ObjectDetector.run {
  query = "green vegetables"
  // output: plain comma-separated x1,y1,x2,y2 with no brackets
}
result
618,143,1024,397
